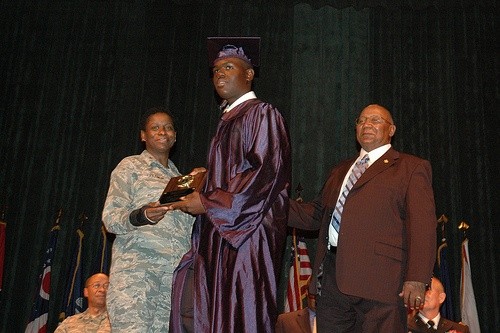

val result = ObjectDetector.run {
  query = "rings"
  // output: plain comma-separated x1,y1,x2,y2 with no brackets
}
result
416,296,421,300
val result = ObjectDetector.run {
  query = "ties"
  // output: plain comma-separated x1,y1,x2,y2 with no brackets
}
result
334,155,368,232
428,320,436,332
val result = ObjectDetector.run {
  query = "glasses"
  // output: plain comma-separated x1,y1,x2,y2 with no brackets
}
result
85,281,109,290
354,114,393,125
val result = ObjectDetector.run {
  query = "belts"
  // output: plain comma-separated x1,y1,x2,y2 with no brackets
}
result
325,245,339,256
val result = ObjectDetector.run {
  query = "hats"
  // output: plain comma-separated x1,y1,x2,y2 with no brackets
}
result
203,33,263,70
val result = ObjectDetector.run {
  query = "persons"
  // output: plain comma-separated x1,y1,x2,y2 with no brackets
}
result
102,110,199,333
54,273,112,333
407,277,470,333
170,36,290,333
288,104,437,333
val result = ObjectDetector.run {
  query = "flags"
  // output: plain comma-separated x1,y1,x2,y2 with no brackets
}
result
0,208,482,333
